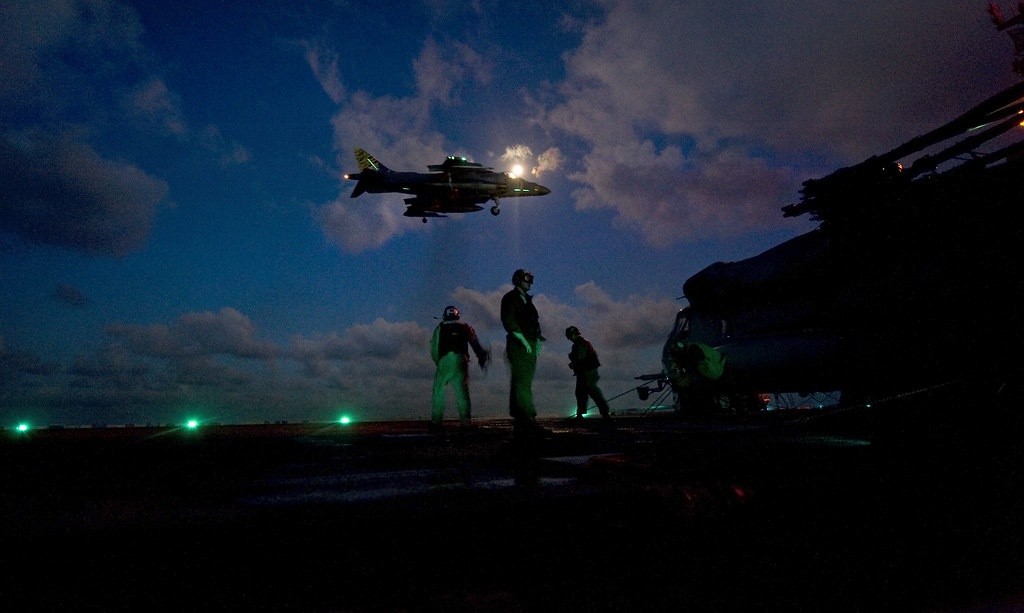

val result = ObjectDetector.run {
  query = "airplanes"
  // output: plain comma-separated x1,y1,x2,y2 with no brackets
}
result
343,146,553,224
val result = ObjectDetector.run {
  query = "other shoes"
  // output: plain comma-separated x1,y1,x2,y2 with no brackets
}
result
514,421,545,433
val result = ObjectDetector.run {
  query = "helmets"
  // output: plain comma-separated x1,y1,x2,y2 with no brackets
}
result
566,325,581,340
443,306,460,320
512,269,532,285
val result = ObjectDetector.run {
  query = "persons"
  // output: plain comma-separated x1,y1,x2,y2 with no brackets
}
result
431,306,487,425
501,269,545,432
671,331,721,432
566,326,611,419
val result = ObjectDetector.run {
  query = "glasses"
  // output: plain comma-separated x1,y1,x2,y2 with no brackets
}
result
513,274,534,283
